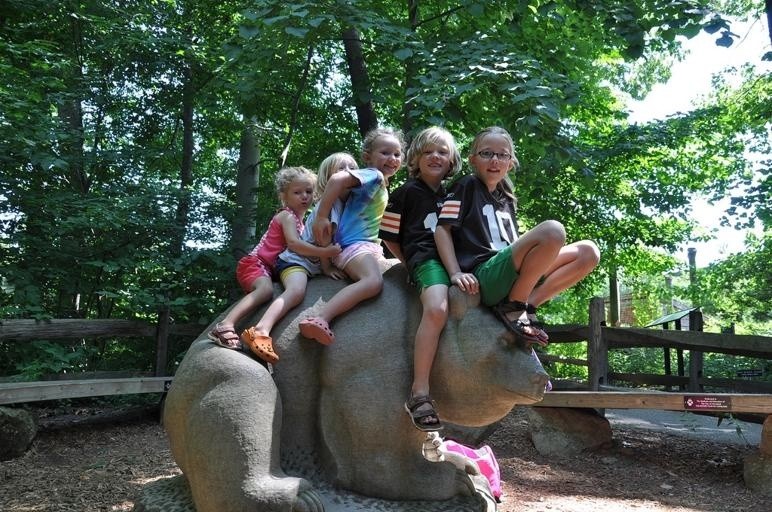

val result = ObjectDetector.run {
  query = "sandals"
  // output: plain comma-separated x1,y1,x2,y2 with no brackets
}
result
404,396,444,432
241,326,279,363
207,323,242,350
491,294,549,347
298,317,335,345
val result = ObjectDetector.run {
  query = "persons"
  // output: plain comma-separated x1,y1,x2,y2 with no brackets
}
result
239,149,362,365
376,124,464,432
432,126,600,348
294,123,406,347
206,165,343,351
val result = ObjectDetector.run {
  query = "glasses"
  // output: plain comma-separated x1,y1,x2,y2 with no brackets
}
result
473,149,512,160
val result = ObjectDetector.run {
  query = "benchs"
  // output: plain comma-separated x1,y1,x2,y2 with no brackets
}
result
0,377,174,405
531,390,772,414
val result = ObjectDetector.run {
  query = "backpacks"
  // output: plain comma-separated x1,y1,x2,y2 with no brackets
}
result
443,439,504,502
